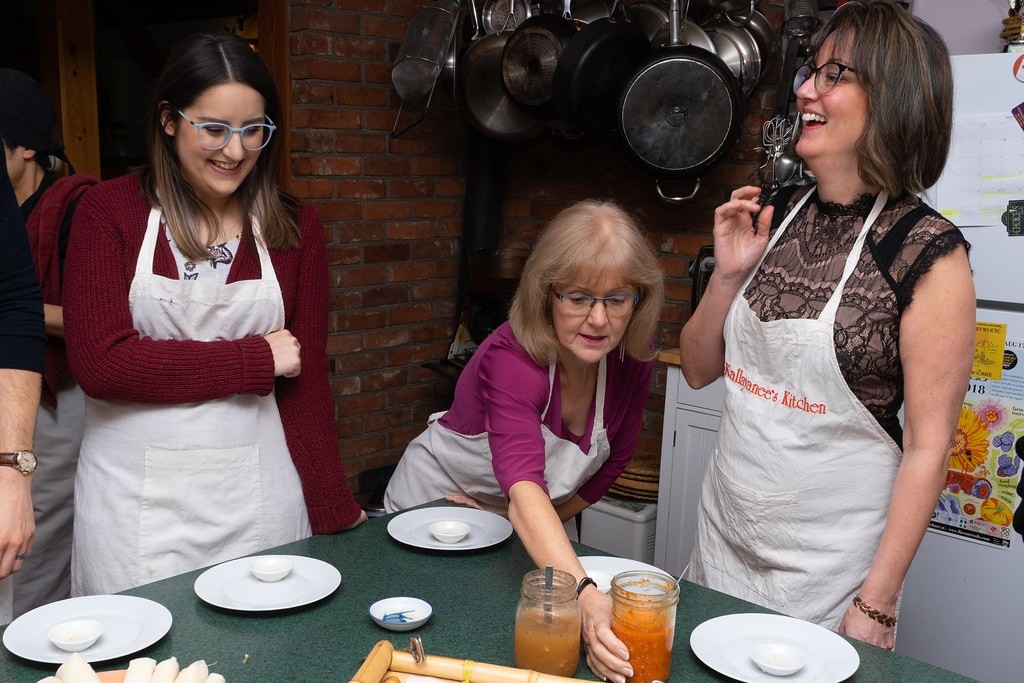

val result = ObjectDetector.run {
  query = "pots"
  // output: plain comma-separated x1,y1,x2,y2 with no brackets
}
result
400,0,778,204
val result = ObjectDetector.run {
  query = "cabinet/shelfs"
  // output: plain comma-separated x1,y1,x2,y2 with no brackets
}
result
654,365,730,582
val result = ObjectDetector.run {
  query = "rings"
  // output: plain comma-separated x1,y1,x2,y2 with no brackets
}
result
17,553,27,560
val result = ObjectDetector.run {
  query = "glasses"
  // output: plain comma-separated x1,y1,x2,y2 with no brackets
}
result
792,56,866,99
550,283,641,316
176,109,278,150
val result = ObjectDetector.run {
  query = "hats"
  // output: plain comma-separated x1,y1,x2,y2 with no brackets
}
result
0,69,56,150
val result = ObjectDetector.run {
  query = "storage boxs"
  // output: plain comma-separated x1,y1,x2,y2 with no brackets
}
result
579,494,658,562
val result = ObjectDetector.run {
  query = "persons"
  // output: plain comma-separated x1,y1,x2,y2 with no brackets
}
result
0,143,44,622
675,2,976,649
63,31,370,599
0,69,104,619
387,201,661,683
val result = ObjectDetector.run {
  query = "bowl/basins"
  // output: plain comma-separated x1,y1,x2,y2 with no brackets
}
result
49,618,102,652
429,520,471,543
751,641,808,676
254,559,295,581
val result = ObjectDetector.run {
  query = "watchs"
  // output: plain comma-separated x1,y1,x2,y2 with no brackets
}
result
0,450,38,474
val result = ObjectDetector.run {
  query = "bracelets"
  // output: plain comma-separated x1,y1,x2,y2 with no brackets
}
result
577,577,597,594
853,594,896,627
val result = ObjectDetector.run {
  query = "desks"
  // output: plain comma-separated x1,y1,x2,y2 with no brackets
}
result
0,499,976,683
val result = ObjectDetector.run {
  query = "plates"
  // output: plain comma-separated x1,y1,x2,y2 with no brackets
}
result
690,613,860,683
193,555,341,611
2,595,173,663
387,506,513,551
369,596,433,632
577,555,675,594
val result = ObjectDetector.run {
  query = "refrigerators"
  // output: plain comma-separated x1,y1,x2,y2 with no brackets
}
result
889,51,1024,681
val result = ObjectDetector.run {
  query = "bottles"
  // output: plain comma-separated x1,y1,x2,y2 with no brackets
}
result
514,568,581,677
612,570,679,682
